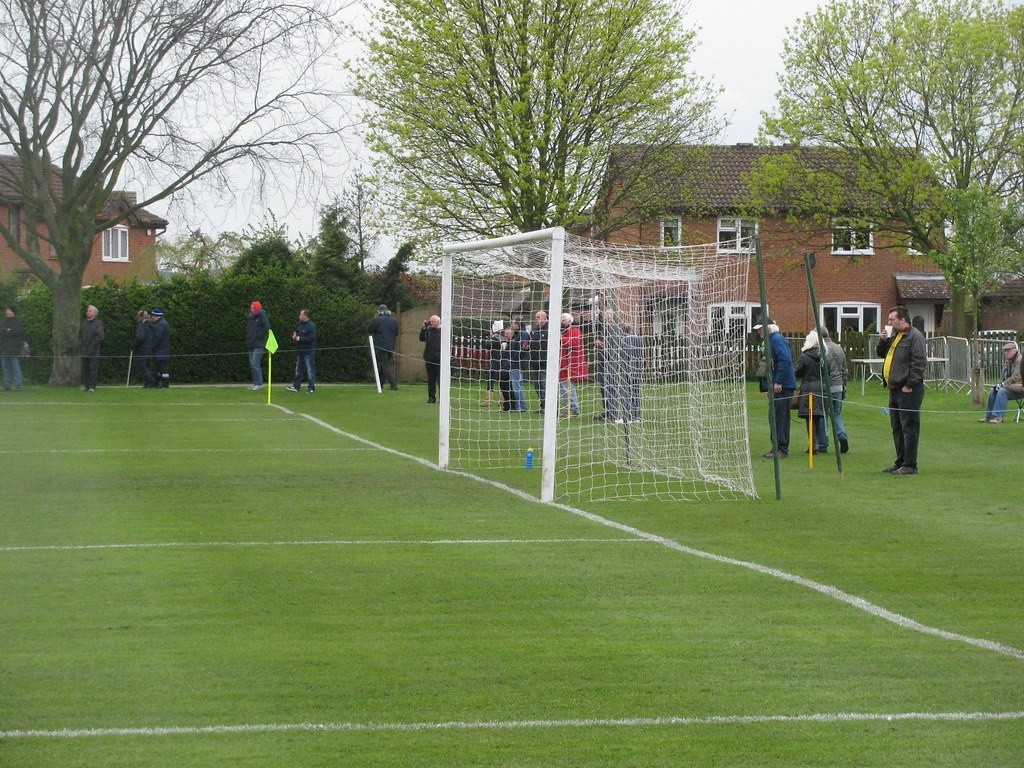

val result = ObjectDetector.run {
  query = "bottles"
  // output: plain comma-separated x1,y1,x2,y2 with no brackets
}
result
525,447,534,469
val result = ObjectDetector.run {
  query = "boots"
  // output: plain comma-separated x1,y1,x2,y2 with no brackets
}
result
480,391,493,407
498,390,504,406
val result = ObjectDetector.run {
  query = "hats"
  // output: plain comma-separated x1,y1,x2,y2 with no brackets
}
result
9,305,16,314
137,311,148,318
752,317,774,329
151,309,163,315
252,302,262,313
1002,343,1016,349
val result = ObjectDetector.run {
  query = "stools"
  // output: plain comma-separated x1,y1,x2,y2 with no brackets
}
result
1010,395,1024,423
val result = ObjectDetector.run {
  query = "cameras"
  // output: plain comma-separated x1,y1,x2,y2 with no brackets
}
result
425,321,431,325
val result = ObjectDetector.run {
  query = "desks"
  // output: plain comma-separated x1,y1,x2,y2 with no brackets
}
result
850,357,950,397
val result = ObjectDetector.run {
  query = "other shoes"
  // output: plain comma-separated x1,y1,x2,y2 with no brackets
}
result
615,418,641,424
761,449,787,459
838,436,849,454
978,417,993,422
560,409,581,416
882,464,916,474
594,411,618,421
248,384,264,390
0,385,11,390
427,396,436,403
286,386,298,393
989,417,1004,424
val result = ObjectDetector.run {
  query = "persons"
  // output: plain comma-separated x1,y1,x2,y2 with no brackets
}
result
528,310,547,415
287,308,315,394
558,313,588,415
0,305,24,392
875,306,926,473
130,309,170,388
245,302,269,391
978,342,1024,423
480,320,506,407
509,316,530,412
793,330,830,454
367,304,398,390
814,325,849,454
616,324,644,424
78,305,104,392
595,309,618,421
419,314,441,403
499,327,515,412
753,317,797,457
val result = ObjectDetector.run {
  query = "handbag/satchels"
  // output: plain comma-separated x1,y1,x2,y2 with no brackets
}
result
759,369,768,392
791,385,801,409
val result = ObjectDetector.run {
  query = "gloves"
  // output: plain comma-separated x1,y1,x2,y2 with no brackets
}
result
993,382,1003,393
